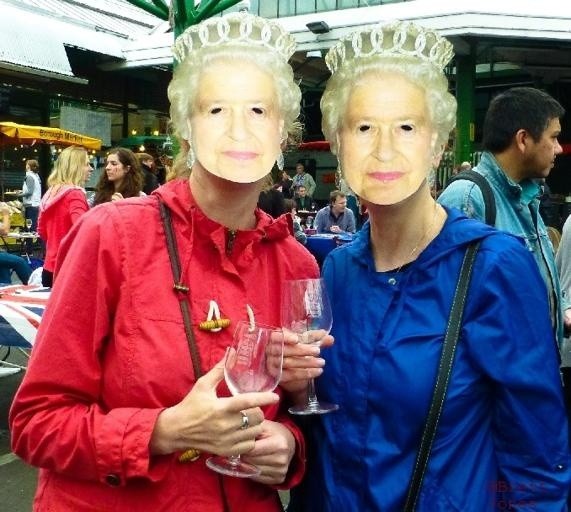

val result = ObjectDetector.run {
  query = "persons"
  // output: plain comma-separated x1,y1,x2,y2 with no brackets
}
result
0,11,571,512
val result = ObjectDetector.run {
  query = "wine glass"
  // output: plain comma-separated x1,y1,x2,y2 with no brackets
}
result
205,321,284,478
279,278,339,415
305,218,319,229
25,219,32,235
